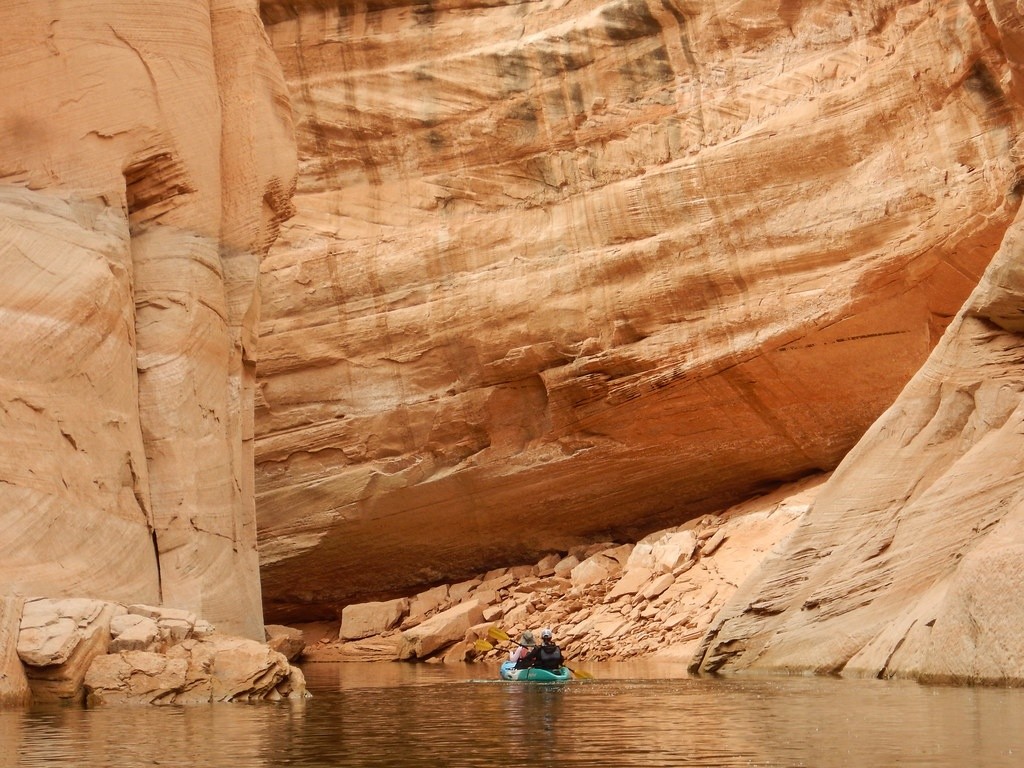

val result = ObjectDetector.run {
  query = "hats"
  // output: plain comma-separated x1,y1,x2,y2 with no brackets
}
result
541,630,552,638
524,630,533,641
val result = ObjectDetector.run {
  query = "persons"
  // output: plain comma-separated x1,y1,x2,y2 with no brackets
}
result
521,629,565,669
509,631,536,666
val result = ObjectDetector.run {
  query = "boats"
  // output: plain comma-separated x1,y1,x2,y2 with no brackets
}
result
499,661,573,682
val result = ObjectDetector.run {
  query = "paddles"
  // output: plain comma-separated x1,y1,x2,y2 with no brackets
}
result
475,639,510,652
487,625,594,679
